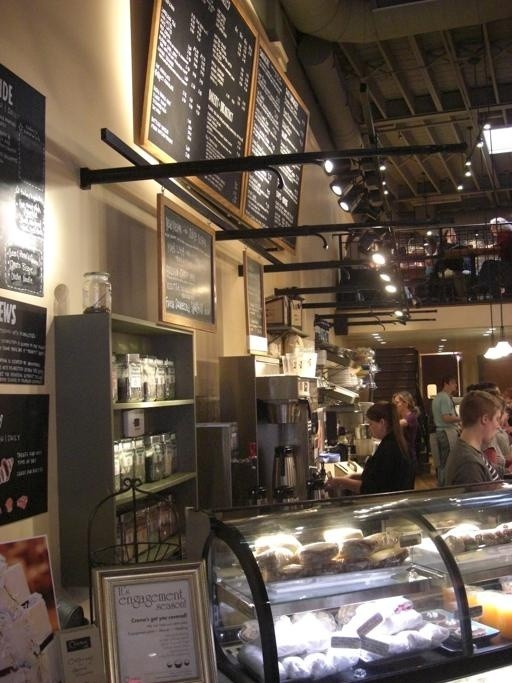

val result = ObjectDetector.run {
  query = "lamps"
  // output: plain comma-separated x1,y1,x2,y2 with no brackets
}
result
325,156,403,318
457,114,491,191
484,304,512,360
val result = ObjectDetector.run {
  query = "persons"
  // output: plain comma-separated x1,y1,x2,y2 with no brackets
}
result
317,396,415,494
341,215,511,306
428,365,463,489
388,388,419,457
443,380,511,487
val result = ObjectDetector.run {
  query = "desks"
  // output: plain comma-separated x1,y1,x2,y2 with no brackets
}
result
449,249,501,303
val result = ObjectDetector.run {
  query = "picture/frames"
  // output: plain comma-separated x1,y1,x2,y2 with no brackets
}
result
89,558,218,682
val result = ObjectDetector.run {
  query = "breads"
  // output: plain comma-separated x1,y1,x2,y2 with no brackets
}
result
443,522,512,556
249,527,410,585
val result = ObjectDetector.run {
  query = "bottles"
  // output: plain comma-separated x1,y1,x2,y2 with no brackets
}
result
82,272,113,314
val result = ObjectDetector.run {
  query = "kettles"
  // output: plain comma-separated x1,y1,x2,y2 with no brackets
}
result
272,444,298,493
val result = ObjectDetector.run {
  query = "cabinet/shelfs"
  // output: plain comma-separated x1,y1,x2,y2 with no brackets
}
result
55,314,200,589
201,476,511,681
